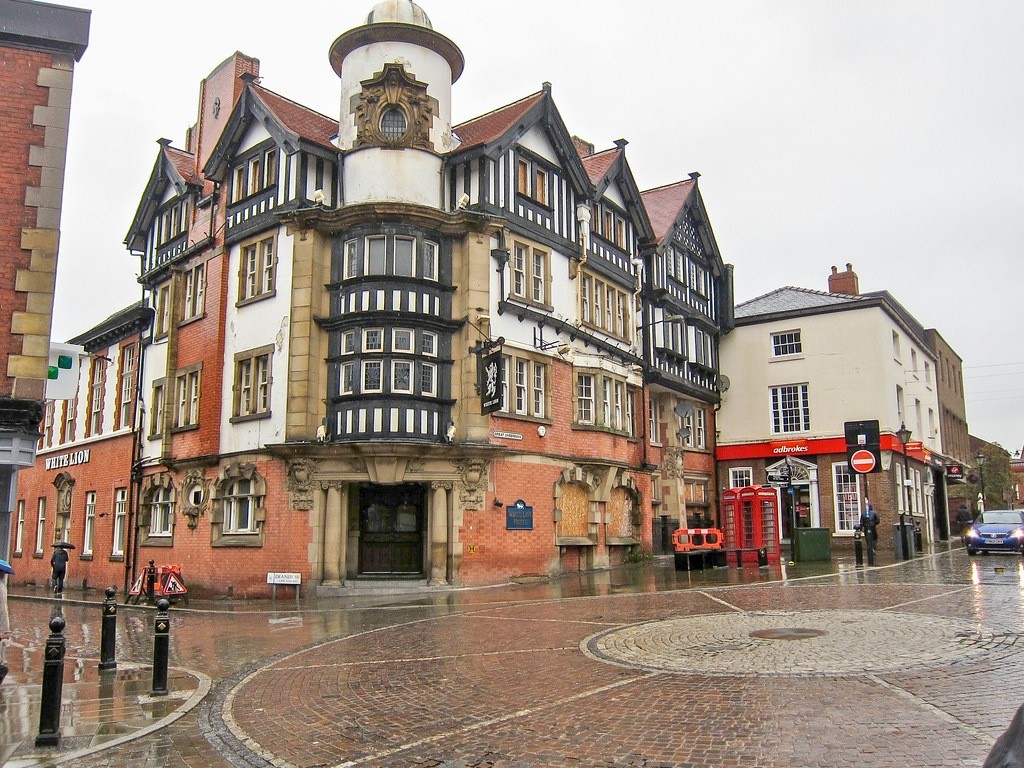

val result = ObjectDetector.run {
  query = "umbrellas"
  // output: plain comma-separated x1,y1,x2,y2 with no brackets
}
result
51,542,76,550
0,559,16,576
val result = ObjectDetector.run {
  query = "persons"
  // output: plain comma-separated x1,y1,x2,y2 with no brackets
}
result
50,547,69,592
0,569,12,685
956,504,971,543
859,504,880,556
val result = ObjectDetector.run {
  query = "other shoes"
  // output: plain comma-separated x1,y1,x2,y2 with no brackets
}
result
56,593,62,595
54,586,57,594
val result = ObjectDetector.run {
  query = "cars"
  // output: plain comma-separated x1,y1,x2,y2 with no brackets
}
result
965,510,1024,557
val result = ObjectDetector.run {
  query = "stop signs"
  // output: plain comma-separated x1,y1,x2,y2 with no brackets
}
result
851,450,876,474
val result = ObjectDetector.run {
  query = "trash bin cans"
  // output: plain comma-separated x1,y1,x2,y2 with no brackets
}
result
893,521,916,559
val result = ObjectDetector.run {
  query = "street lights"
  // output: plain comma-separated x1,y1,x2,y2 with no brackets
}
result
897,423,913,527
976,452,988,510
